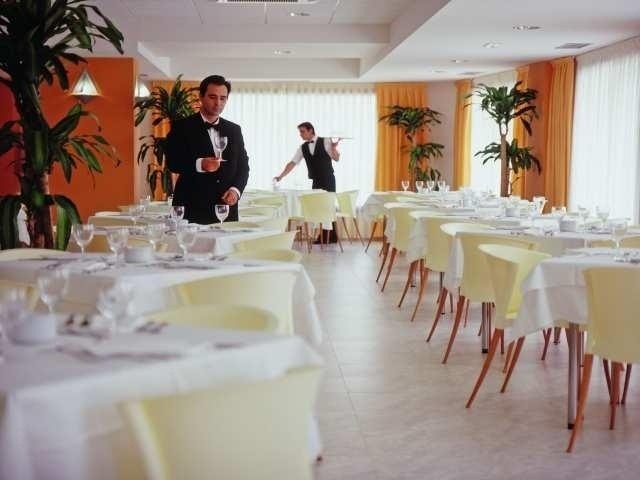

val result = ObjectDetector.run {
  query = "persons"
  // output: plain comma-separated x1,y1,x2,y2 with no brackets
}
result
271,122,342,244
164,75,250,226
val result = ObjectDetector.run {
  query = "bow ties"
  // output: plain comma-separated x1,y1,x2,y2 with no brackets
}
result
305,140,314,144
203,121,220,131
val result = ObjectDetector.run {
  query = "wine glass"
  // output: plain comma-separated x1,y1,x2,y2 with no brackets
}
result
1,195,230,343
401,179,639,262
215,137,228,162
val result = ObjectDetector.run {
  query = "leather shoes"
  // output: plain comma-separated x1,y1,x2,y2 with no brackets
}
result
312,238,338,243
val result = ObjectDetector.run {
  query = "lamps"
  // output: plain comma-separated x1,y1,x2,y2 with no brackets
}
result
67,69,100,106
132,75,154,108
